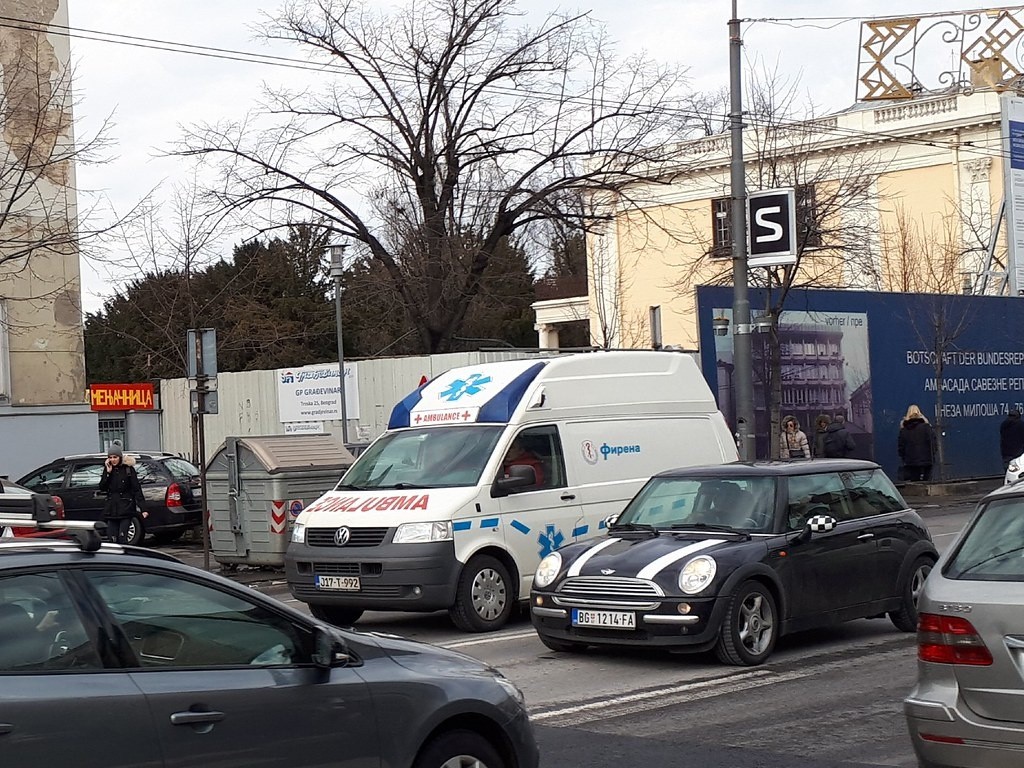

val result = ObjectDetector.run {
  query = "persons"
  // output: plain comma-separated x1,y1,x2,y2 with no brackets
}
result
683,483,740,523
36,611,59,631
99,440,148,544
779,415,810,459
1000,410,1024,486
506,437,544,487
812,415,856,458
898,405,938,480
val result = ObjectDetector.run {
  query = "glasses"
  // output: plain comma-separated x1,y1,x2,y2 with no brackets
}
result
785,423,793,428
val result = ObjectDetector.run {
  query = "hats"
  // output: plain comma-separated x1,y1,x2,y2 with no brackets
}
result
108,439,123,458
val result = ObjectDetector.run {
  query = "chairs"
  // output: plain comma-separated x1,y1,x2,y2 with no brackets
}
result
721,484,755,527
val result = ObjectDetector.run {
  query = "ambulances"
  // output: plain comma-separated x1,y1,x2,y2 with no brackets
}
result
284,351,747,629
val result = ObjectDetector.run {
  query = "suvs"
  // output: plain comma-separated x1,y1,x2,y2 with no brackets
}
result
16,450,202,546
901,477,1024,767
0,537,542,768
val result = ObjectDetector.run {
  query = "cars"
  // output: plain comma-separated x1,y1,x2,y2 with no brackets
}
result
531,456,938,666
1005,450,1024,485
0,477,67,536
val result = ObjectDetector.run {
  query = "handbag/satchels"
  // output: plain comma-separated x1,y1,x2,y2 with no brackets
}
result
785,433,806,460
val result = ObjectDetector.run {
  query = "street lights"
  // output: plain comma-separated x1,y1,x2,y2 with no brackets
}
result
323,242,351,449
